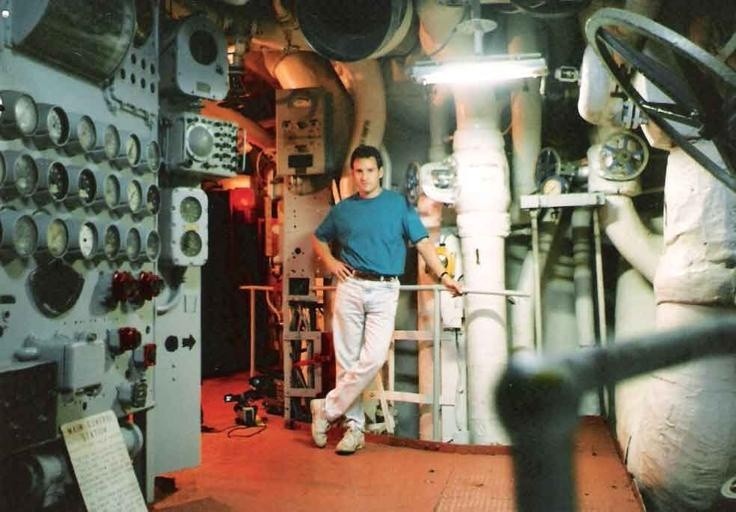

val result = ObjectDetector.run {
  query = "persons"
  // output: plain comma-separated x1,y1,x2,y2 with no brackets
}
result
309,145,463,454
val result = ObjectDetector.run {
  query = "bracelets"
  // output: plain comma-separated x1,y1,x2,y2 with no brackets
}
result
438,271,447,281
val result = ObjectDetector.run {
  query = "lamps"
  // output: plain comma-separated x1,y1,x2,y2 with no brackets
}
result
412,30,549,88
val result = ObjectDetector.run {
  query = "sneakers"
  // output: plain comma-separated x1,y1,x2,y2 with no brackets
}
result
334,420,366,454
309,398,333,449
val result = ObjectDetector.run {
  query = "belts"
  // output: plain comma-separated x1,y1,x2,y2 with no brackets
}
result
344,268,398,282
